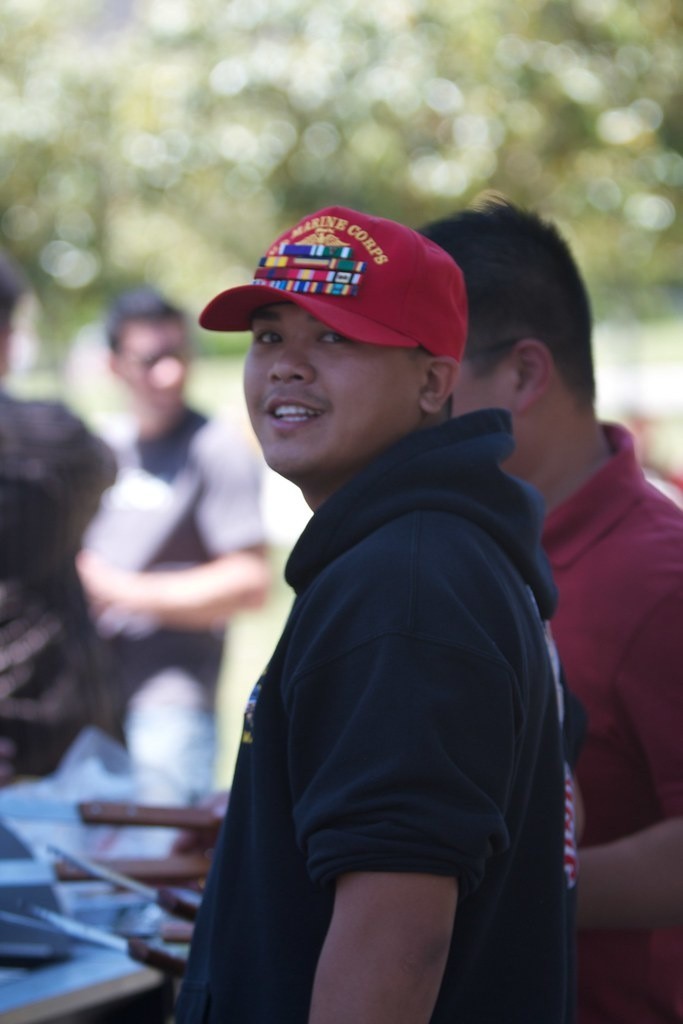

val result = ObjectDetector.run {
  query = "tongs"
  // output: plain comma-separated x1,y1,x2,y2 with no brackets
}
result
31,843,200,979
53,802,223,882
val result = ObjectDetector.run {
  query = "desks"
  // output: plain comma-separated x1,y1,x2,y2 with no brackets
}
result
0,766,205,1024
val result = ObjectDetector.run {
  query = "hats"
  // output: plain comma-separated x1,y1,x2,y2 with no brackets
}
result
198,207,466,366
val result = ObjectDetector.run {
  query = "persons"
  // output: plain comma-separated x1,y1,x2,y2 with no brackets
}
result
0,262,127,790
76,289,272,793
173,206,581,1024
419,203,683,1024
